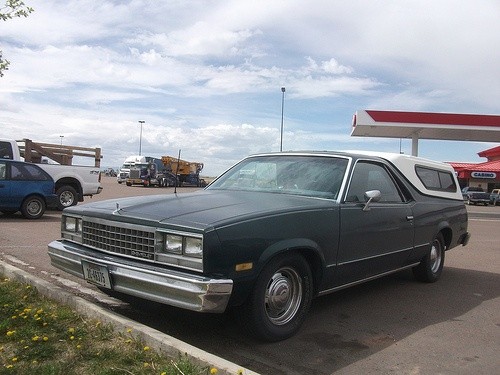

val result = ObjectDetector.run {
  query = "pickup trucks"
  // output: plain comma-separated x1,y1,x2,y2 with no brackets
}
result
0,138,103,212
461,186,491,206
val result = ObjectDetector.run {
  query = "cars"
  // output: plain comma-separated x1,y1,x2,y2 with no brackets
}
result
0,158,59,221
45,148,473,343
490,188,500,206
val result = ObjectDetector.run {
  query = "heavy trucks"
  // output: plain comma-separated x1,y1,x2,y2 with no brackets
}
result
125,155,205,188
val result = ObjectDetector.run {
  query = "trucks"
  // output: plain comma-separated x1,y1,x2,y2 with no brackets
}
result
117,155,164,185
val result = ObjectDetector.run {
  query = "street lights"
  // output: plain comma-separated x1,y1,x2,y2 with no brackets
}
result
137,120,145,155
280,86,286,152
